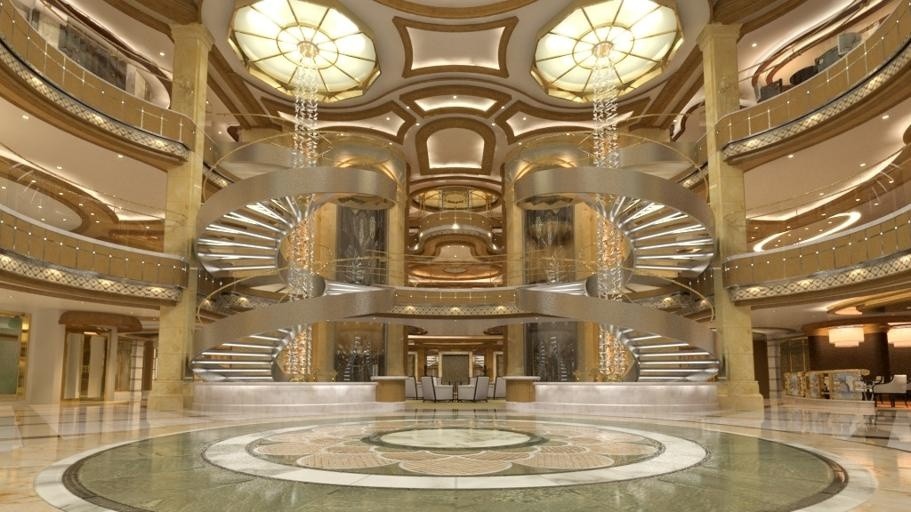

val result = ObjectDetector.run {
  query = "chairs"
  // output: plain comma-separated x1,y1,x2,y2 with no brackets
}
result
756,31,863,103
406,375,507,403
823,374,909,408
